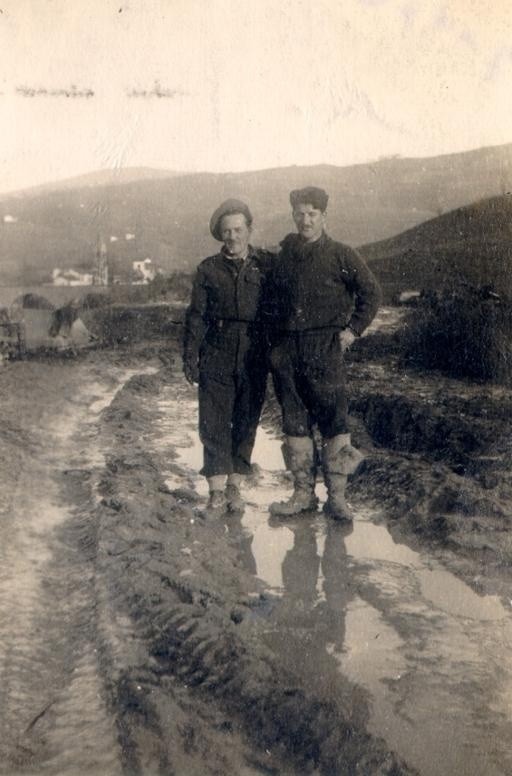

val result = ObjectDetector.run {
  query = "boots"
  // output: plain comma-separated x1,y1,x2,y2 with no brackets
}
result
268,443,320,517
319,441,365,523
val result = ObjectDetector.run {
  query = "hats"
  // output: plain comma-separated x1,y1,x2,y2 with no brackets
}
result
209,199,252,242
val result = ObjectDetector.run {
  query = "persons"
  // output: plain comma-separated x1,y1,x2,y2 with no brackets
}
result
182,199,281,522
268,509,351,646
266,185,383,521
205,513,258,576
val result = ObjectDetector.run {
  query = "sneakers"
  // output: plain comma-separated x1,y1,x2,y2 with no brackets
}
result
227,485,245,514
195,490,228,522
